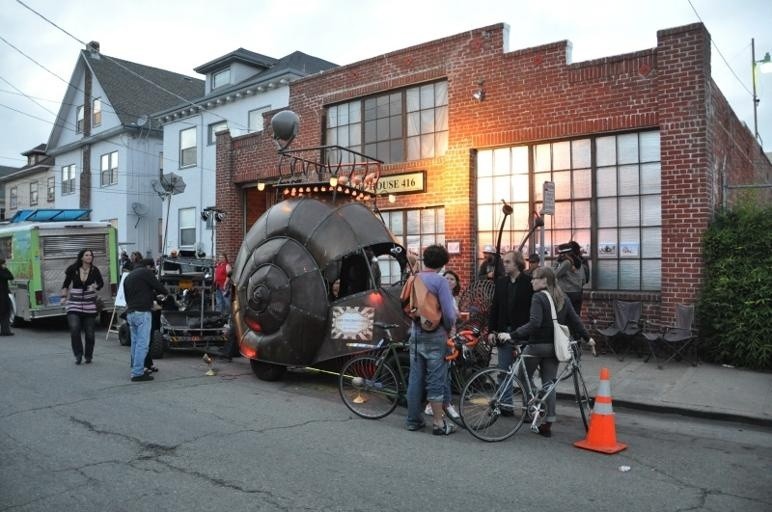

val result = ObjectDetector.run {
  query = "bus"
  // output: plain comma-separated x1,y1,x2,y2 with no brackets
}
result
0,207,121,331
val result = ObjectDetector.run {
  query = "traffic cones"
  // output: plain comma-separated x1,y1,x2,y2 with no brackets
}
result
574,368,629,455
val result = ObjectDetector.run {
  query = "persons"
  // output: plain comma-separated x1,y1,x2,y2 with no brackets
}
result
459,261,496,393
123,257,168,382
487,250,534,417
567,241,590,284
60,248,104,365
424,270,460,419
480,245,505,277
551,243,587,359
524,253,541,274
131,289,161,374
134,253,144,264
0,259,16,337
399,243,458,435
498,267,598,439
119,253,131,276
213,252,232,313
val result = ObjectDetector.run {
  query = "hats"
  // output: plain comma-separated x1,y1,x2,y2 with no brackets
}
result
558,244,572,252
143,249,155,267
482,245,496,254
525,254,540,261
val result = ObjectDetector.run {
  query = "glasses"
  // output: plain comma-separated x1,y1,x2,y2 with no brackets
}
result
529,261,538,263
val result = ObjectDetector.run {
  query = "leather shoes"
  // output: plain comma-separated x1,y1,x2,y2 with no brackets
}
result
4,332,14,336
534,425,552,437
75,357,83,365
0,332,3,336
86,358,91,364
132,374,153,381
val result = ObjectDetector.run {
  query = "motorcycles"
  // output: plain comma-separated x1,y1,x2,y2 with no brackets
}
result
117,254,232,360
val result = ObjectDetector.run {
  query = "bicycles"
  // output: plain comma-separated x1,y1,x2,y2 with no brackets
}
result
339,319,502,431
458,338,594,443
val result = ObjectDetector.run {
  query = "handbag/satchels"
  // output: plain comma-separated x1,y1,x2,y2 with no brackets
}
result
541,290,572,361
399,276,444,332
91,266,106,313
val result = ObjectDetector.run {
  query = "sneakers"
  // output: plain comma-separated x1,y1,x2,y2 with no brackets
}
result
143,367,153,374
149,365,159,372
488,405,514,417
443,404,460,418
424,404,435,416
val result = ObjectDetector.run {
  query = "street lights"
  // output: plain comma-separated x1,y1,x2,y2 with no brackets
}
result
751,37,772,138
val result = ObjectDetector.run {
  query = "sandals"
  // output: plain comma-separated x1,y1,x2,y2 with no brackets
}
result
433,420,458,436
407,422,426,431
